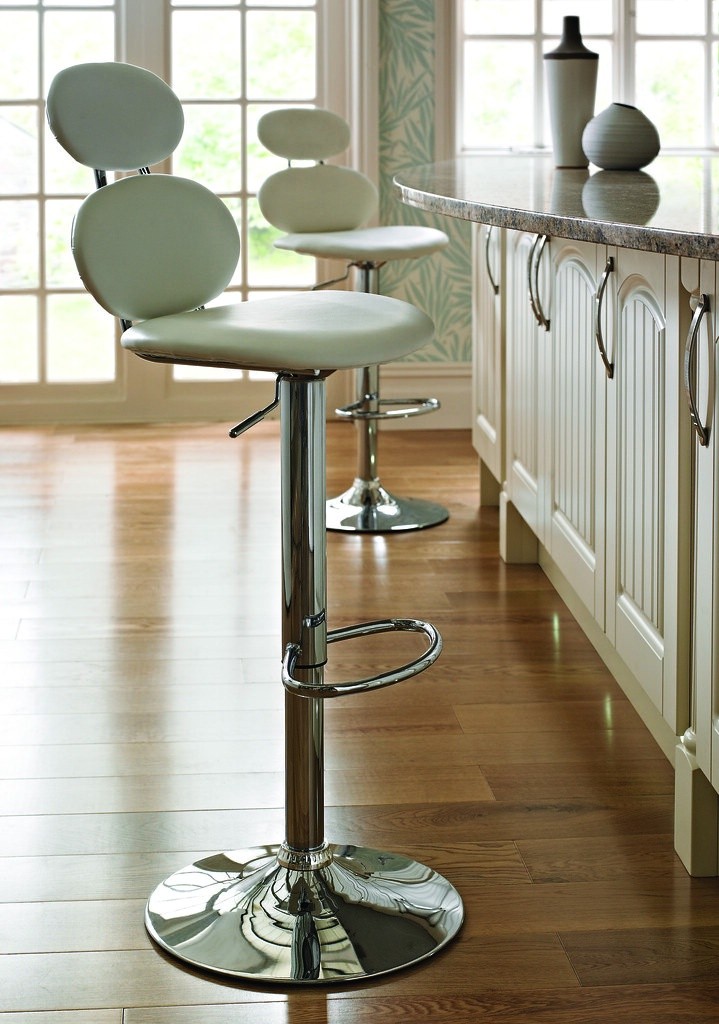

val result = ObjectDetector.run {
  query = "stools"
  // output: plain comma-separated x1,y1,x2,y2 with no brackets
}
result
48,61,463,990
256,104,452,536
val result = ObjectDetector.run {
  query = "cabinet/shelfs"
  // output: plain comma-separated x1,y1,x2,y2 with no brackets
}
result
471,222,719,879
